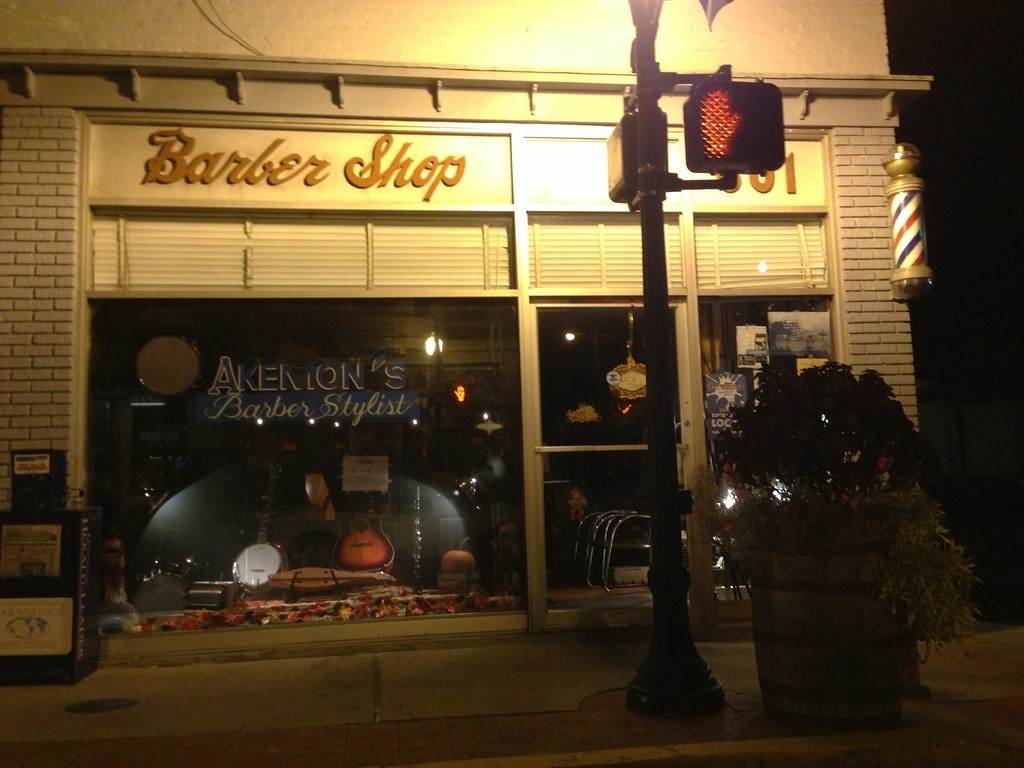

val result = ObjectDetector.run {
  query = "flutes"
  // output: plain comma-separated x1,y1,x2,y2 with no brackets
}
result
412,485,422,594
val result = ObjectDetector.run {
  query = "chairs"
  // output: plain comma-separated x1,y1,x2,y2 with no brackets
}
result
571,480,655,594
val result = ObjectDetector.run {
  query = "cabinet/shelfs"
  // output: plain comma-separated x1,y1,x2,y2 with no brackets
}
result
0,506,104,687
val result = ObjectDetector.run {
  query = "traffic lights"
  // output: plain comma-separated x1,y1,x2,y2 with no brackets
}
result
684,64,787,178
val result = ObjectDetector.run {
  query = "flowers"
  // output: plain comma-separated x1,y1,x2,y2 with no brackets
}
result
692,441,983,662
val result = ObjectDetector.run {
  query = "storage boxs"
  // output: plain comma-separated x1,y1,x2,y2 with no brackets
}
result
438,572,480,595
608,564,649,585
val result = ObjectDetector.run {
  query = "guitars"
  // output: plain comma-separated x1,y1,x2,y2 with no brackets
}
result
331,505,396,575
232,460,289,597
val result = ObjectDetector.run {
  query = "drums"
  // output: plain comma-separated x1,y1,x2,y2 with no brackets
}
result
133,570,187,611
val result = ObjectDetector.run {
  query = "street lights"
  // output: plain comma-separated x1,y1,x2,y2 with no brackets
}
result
421,330,443,486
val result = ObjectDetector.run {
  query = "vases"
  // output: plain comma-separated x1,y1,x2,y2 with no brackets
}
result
746,535,908,724
